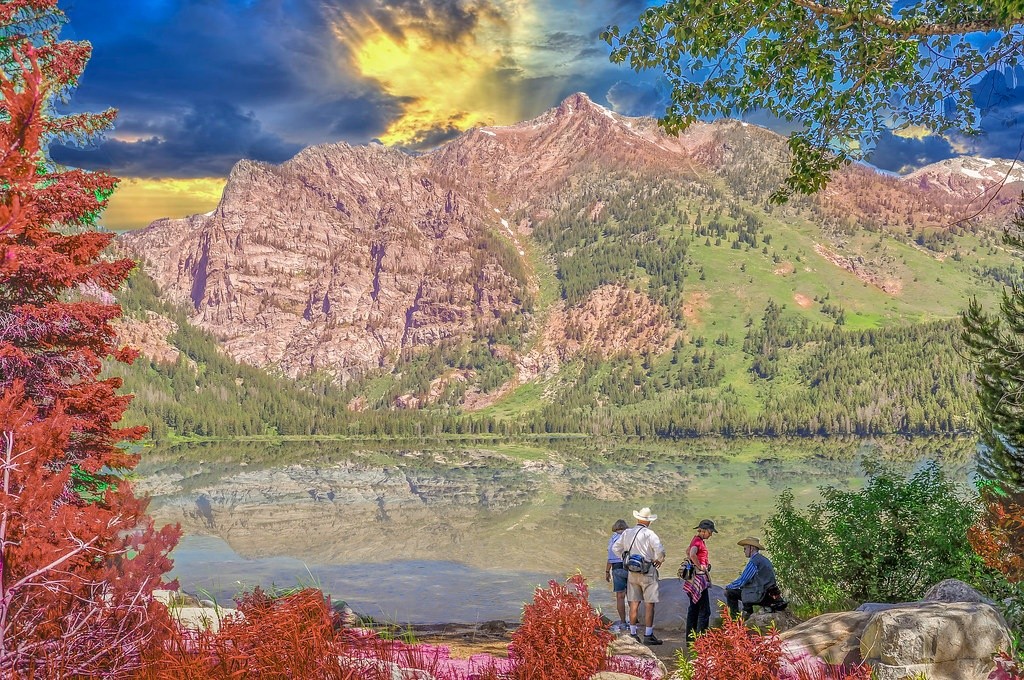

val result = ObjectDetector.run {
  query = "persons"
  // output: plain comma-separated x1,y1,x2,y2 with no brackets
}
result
605,508,665,645
683,519,718,647
723,537,776,620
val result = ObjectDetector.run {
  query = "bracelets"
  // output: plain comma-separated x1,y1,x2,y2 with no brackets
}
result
606,570,608,572
701,565,704,569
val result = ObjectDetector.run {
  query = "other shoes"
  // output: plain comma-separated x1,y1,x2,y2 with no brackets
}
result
643,634,662,645
741,611,751,621
621,622,626,630
631,634,641,643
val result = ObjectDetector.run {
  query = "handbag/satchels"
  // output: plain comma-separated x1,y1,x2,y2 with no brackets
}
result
677,559,694,580
761,586,788,611
626,554,651,575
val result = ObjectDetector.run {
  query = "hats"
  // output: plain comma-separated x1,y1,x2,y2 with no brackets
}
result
699,519,718,533
632,507,657,521
737,537,765,550
